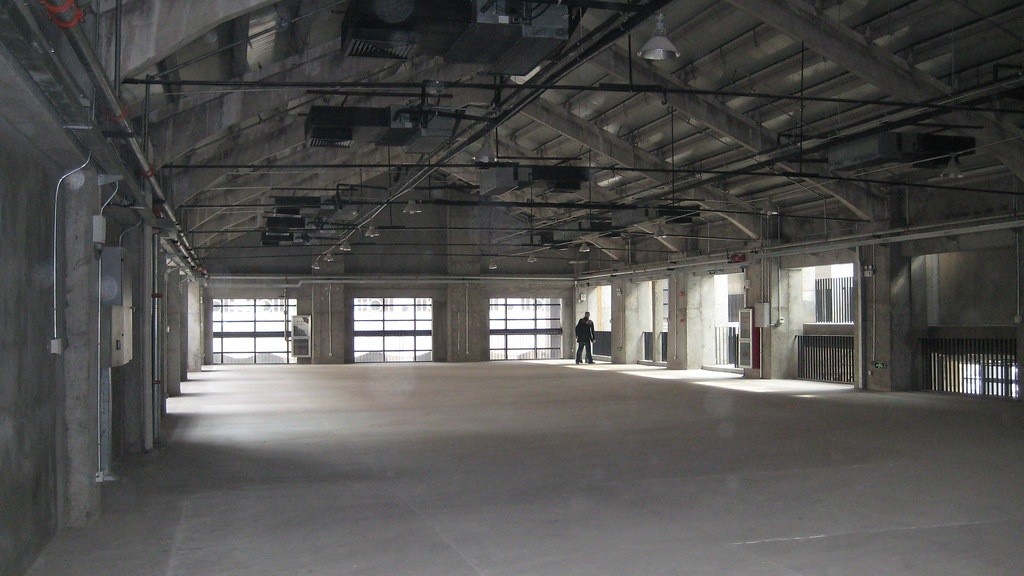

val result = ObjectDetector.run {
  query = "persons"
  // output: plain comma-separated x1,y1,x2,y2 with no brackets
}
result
576,317,595,364
578,312,596,363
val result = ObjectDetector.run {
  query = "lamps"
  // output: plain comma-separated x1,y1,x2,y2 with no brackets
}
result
489,258,497,270
475,136,495,162
636,12,680,60
322,252,334,262
616,288,622,295
339,240,351,252
940,154,964,179
579,242,590,252
401,200,422,214
364,219,379,237
760,198,780,214
654,224,666,238
311,260,320,269
863,264,873,277
527,253,537,262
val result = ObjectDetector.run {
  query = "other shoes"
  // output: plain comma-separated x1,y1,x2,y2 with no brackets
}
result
588,362,596,365
576,360,584,364
